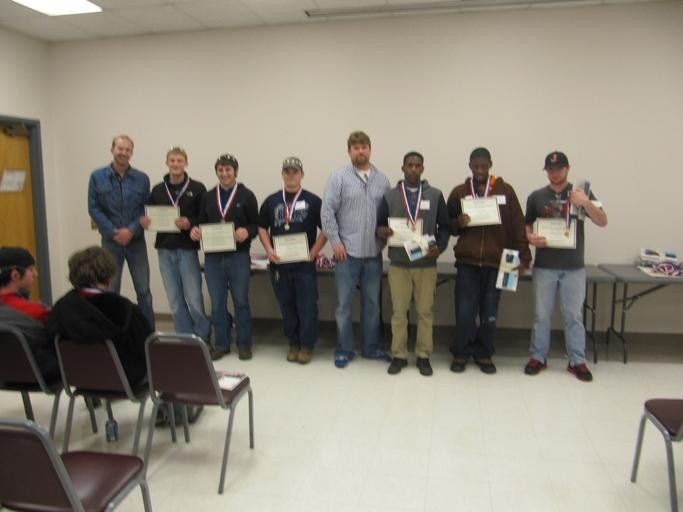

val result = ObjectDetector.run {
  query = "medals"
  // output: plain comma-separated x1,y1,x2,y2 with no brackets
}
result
564,231,571,237
284,224,290,231
220,219,225,224
411,226,416,231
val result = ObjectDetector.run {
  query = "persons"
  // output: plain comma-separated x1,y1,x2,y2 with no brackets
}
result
0,247,51,325
188,154,258,361
321,131,392,369
43,246,155,398
447,148,531,373
375,152,447,377
88,135,155,336
524,152,607,381
141,149,213,354
258,157,326,363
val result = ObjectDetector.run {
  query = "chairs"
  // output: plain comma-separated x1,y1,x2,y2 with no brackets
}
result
54,333,175,457
0,418,152,512
630,398,683,512
143,331,255,494
0,325,98,439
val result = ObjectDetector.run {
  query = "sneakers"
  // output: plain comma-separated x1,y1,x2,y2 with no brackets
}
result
474,357,496,373
524,358,548,375
335,352,349,368
287,344,312,364
362,351,388,360
416,357,432,376
450,357,468,373
567,361,592,381
211,346,229,360
387,357,408,374
238,342,252,359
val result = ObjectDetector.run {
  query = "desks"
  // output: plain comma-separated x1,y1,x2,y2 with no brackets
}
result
384,259,619,364
597,263,683,363
201,251,385,338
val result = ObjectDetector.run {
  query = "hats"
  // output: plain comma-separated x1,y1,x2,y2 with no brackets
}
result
215,155,238,169
283,158,303,171
545,152,568,169
